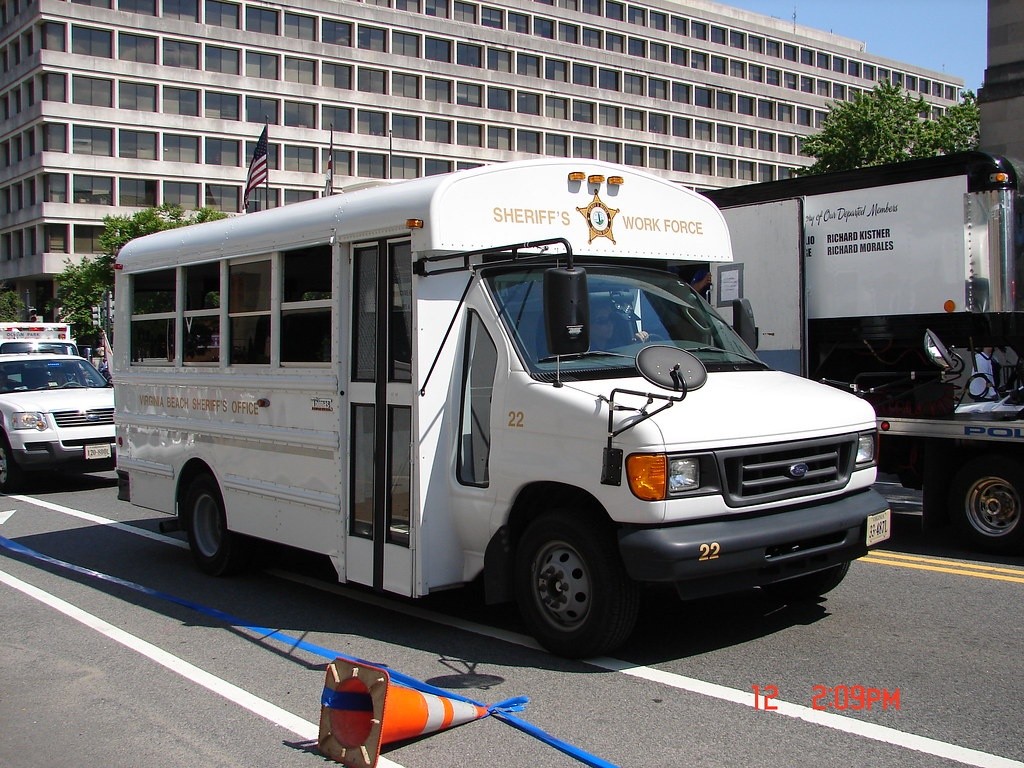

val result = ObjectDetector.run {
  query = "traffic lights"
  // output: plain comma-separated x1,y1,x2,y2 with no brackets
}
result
108,291,115,318
28,306,36,322
91,303,101,328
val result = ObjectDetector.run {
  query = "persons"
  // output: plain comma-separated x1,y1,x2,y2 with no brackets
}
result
692,272,711,295
971,346,998,402
592,304,649,350
29,310,112,386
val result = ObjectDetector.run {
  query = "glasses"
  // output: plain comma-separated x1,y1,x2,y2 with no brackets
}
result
594,312,617,325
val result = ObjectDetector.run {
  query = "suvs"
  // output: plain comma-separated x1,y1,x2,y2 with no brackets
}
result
0,351,116,494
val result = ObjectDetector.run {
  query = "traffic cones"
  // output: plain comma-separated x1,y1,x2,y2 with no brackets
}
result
315,658,489,768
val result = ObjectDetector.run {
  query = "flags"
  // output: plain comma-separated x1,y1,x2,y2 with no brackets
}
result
324,134,331,196
242,123,268,209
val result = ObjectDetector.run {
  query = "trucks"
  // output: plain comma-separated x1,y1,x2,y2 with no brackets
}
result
697,151,1024,557
114,158,893,662
0,322,80,370
